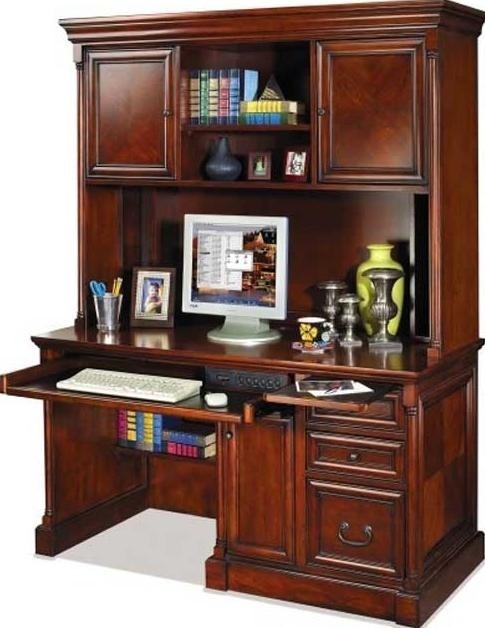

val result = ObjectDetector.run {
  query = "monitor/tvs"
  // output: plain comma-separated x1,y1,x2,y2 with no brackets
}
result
181,214,288,346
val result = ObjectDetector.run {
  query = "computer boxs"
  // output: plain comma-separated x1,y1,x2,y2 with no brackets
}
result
205,365,292,394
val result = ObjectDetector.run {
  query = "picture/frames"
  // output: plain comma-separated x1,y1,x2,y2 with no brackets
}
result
247,150,271,180
129,266,176,328
282,147,309,182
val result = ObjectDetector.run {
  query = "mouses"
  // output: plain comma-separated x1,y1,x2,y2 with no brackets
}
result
203,391,229,406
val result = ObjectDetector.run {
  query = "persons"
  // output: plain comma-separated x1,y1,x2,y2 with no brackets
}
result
144,283,162,314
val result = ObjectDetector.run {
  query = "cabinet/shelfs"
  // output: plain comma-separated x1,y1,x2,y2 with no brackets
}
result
0,0,485,628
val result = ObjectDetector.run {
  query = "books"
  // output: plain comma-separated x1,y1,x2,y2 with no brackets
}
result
190,68,307,125
116,411,216,458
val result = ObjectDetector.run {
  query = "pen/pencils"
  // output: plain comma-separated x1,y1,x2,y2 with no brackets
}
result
112,277,124,296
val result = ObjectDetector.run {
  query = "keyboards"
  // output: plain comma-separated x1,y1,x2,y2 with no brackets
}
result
54,367,203,404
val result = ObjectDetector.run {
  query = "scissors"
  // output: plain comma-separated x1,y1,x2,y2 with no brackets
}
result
89,279,107,296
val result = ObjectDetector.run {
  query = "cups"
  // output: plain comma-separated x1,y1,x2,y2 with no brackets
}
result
296,316,335,351
94,293,123,332
